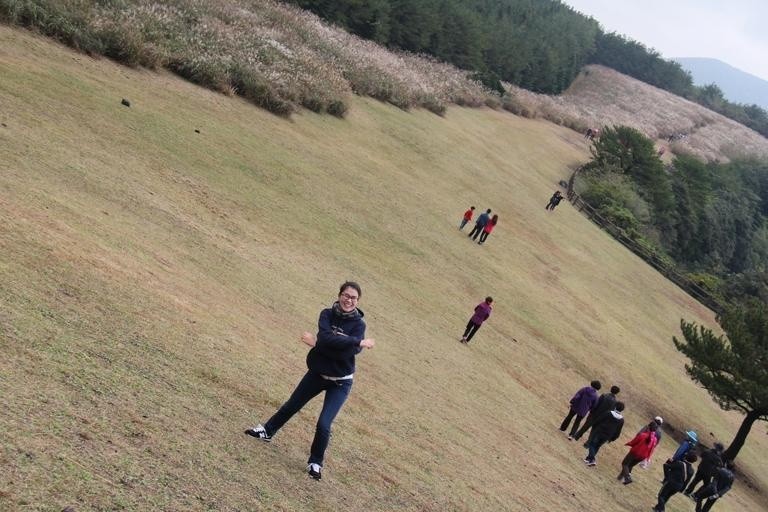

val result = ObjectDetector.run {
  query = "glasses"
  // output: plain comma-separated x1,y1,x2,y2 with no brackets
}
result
342,293,358,300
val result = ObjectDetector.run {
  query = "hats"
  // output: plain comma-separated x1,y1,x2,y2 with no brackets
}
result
655,415,663,424
686,429,698,442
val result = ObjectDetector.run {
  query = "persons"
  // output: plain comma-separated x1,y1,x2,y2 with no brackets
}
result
658,133,674,158
459,205,476,231
459,297,493,345
468,208,492,241
584,128,600,142
241,279,374,482
478,212,498,245
558,379,735,512
545,191,563,212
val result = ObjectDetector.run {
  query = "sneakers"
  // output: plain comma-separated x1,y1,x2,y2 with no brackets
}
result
244,424,272,442
586,459,596,466
306,461,323,481
623,477,632,484
